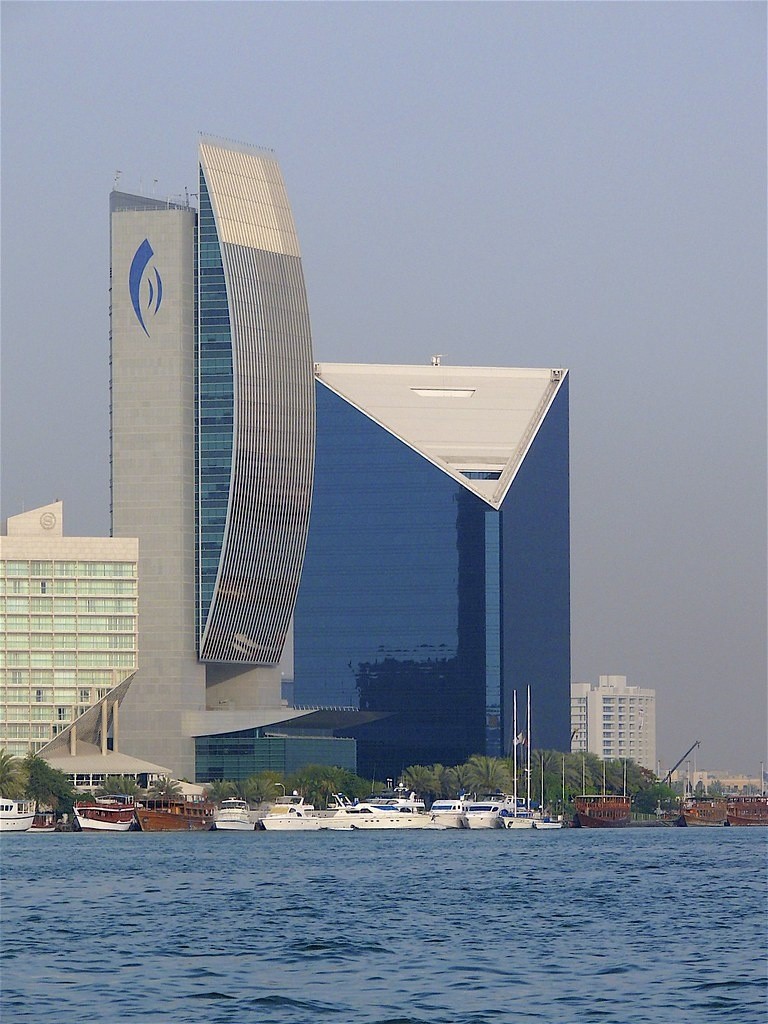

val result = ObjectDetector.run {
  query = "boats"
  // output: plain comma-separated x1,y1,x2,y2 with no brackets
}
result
501,816,535,831
73,793,135,831
27,814,55,832
681,796,729,827
533,821,562,829
468,788,525,830
319,792,431,830
576,796,631,828
258,805,321,831
727,794,768,827
430,791,474,828
214,797,255,830
0,796,36,833
134,794,217,832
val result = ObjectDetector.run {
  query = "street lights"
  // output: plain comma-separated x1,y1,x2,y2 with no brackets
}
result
274,783,285,797
